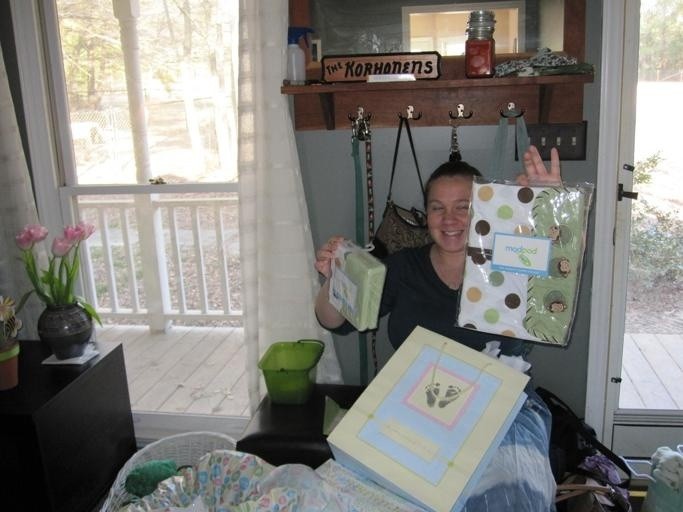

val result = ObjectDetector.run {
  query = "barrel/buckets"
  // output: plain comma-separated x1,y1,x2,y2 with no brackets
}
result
258,339,325,406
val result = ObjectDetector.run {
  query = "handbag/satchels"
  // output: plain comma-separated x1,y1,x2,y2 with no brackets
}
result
327,324,533,507
370,115,432,259
532,385,634,489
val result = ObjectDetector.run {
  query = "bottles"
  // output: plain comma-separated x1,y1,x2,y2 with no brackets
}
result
465,11,496,78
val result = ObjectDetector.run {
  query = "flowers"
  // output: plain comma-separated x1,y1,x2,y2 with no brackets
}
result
13,222,103,329
0,296,23,344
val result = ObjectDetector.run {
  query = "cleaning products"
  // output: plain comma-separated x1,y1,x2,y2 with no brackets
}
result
288,26,314,82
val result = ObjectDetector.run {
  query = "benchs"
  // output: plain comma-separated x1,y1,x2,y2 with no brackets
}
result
236,386,367,466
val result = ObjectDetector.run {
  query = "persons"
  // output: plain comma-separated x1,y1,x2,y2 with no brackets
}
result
313,143,562,366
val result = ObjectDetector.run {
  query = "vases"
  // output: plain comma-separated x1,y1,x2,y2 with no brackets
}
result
0,343,19,391
37,301,93,359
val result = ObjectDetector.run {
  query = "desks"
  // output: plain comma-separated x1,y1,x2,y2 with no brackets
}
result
0,339,139,511
